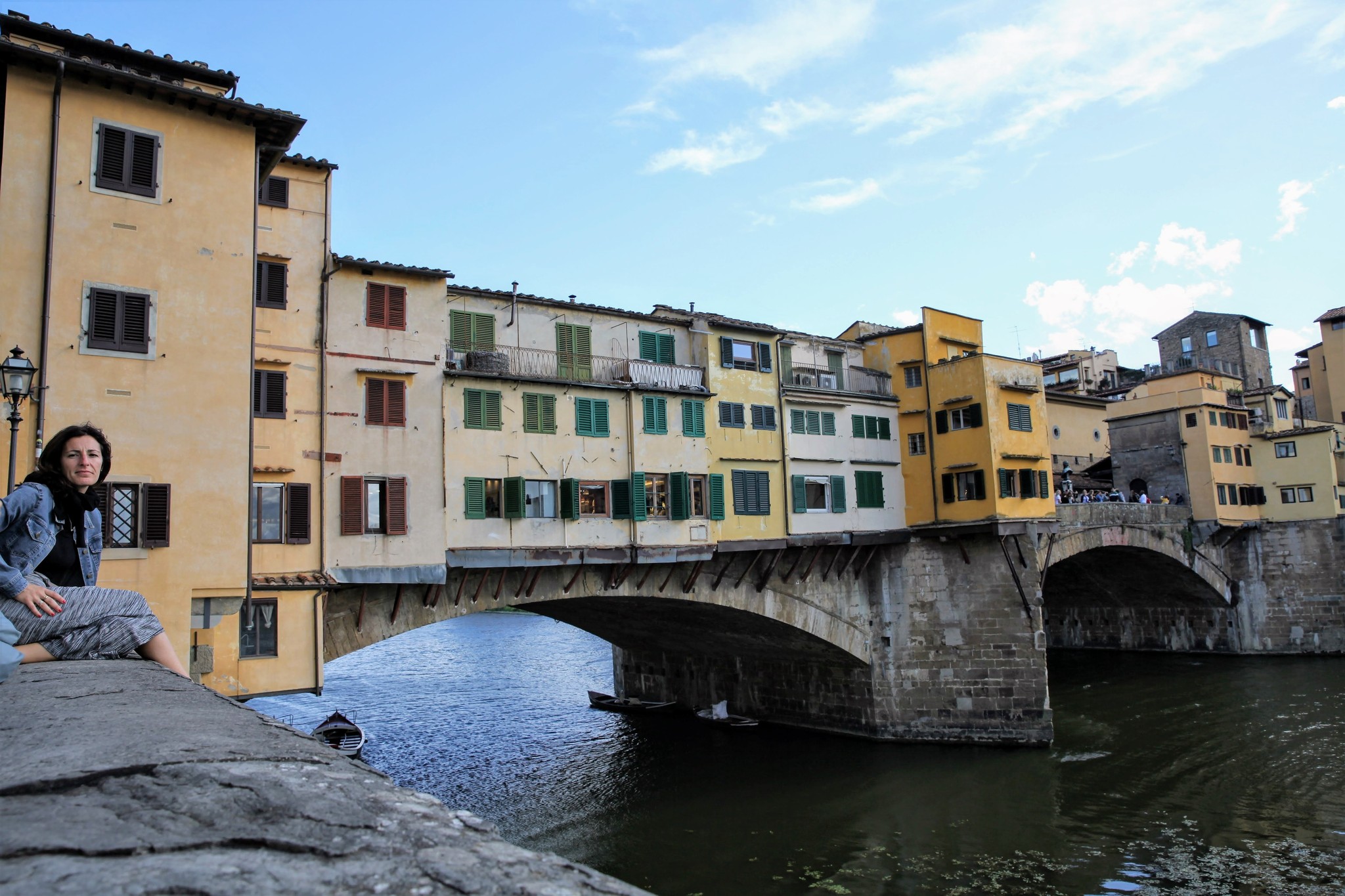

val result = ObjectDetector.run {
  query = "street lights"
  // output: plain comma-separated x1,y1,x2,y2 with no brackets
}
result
0,346,40,499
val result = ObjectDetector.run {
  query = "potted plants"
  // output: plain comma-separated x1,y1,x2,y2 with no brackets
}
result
1226,388,1243,397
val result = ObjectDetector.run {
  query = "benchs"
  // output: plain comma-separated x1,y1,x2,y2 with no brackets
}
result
340,735,361,747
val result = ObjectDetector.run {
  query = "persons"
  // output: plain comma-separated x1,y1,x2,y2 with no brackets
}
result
1054,488,1185,506
650,498,700,516
485,496,499,518
0,421,195,683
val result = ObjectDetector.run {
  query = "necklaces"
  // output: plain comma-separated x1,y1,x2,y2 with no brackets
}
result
67,513,73,532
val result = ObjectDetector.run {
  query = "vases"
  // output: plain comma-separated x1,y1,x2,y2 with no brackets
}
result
1199,363,1204,367
1210,366,1215,369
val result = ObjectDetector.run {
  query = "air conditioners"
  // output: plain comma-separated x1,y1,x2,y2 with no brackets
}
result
796,374,816,387
1248,407,1262,424
818,374,837,389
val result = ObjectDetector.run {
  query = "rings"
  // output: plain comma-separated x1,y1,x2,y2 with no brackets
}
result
37,601,44,606
43,596,48,600
